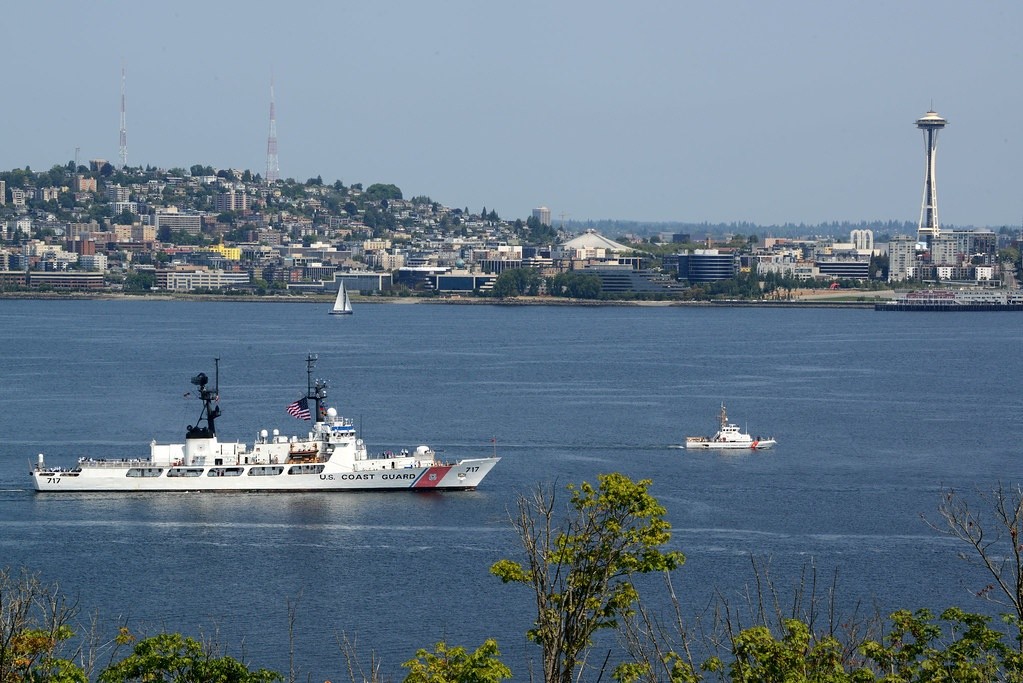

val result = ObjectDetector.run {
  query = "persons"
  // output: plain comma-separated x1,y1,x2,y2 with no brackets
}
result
383,448,408,458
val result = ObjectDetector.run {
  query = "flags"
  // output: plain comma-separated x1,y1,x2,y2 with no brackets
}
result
285,396,311,420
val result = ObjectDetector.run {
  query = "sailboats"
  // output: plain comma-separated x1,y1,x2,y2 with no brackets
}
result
327,276,354,314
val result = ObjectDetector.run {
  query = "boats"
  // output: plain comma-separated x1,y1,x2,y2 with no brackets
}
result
684,400,777,450
874,275,1023,311
27,349,503,492
